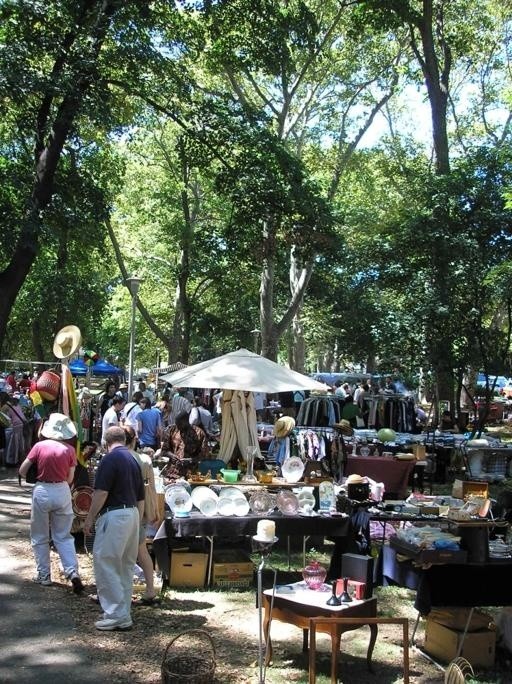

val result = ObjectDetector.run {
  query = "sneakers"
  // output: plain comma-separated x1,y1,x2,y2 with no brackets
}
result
33,576,52,586
71,572,84,594
95,617,133,631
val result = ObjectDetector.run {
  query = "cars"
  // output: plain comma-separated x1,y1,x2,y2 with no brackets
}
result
469,375,512,424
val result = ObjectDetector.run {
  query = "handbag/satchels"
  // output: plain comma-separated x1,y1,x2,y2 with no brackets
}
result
142,478,159,522
22,421,31,437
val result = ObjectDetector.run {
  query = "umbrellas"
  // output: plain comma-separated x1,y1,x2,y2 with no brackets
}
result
159,347,331,395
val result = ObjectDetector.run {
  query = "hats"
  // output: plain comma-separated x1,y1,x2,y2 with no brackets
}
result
52,324,83,359
273,415,296,438
332,418,354,437
41,412,78,440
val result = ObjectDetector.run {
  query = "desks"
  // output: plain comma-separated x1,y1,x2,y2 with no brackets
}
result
260,580,378,683
185,475,306,491
410,556,512,674
345,432,512,486
348,454,416,499
338,499,505,588
170,509,351,587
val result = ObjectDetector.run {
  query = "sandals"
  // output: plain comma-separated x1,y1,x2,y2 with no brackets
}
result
130,592,160,605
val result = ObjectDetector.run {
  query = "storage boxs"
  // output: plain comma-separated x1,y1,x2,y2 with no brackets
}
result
423,616,496,668
170,537,209,587
211,546,254,586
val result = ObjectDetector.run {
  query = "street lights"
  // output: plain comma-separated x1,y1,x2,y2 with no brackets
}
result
125,270,145,402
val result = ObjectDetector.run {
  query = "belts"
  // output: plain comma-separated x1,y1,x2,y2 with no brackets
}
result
38,479,66,484
97,504,137,516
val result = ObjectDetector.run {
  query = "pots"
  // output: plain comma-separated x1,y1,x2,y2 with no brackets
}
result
220,468,241,482
255,469,277,483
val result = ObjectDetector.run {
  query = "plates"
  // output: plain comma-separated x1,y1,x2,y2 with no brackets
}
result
281,456,305,484
247,490,299,517
164,484,251,518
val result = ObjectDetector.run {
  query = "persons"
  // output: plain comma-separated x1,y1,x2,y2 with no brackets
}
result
415,406,427,424
393,376,407,394
119,425,159,605
81,426,147,631
103,378,223,482
278,376,396,419
19,413,83,595
0,366,46,466
